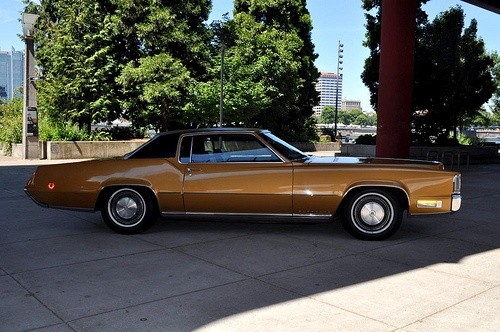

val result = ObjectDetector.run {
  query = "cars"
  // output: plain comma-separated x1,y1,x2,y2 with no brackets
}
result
24,127,462,240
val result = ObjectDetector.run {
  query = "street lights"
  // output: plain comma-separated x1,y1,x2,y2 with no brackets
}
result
18,12,40,159
